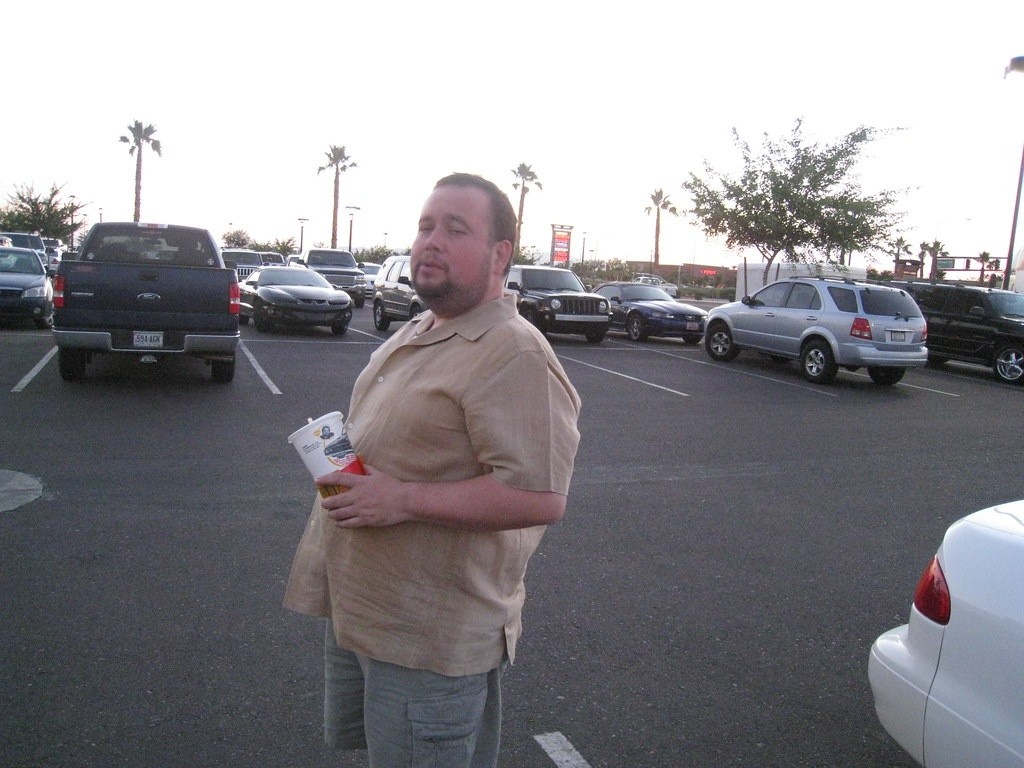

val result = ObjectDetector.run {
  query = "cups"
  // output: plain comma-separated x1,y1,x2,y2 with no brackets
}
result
287,411,367,511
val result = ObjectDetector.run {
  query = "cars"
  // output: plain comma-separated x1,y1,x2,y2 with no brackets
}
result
868,500,1023,768
224,248,389,308
592,279,708,342
238,265,353,333
1,248,50,330
632,273,678,297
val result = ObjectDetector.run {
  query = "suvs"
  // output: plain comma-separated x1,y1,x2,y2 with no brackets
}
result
370,253,423,331
706,276,928,385
1,232,67,276
506,262,613,344
882,280,1024,384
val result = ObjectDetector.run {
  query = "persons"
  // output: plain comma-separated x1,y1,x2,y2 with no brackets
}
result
285,173,581,768
320,426,334,439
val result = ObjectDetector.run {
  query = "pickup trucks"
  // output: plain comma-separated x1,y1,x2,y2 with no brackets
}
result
49,219,240,382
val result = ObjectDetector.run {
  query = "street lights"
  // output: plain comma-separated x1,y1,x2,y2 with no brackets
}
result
346,206,361,251
297,218,309,254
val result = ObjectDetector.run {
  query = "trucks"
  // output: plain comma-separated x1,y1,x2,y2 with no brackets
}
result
735,263,868,305
